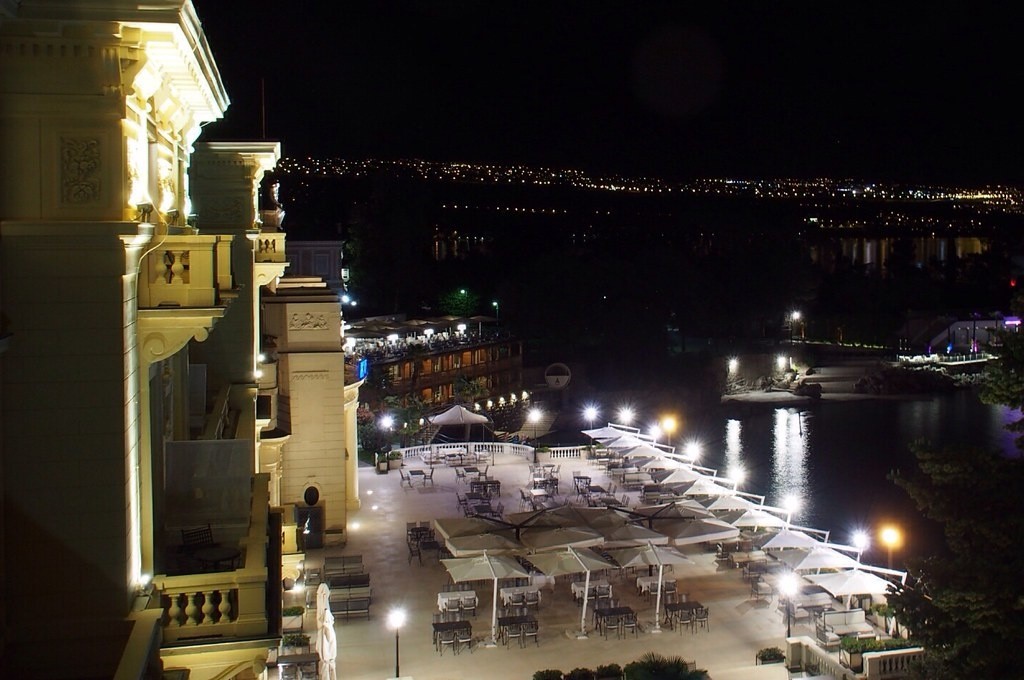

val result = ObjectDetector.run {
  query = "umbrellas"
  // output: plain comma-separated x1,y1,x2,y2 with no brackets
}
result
441,552,531,582
698,494,762,509
714,510,792,527
634,500,741,545
525,546,620,578
315,583,331,629
582,427,730,495
768,548,863,568
606,541,696,567
803,570,903,596
316,607,337,680
434,506,669,556
742,530,827,548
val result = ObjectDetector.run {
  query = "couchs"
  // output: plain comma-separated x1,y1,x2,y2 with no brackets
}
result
438,447,467,458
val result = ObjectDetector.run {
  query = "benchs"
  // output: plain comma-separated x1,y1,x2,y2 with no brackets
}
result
622,473,655,492
610,467,640,480
716,538,877,653
304,554,373,621
595,449,608,459
607,459,636,472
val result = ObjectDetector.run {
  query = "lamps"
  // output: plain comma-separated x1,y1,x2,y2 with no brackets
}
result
167,209,179,226
189,214,200,228
136,204,153,223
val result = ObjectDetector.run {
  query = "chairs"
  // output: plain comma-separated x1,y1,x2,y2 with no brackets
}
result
398,460,710,657
181,524,220,575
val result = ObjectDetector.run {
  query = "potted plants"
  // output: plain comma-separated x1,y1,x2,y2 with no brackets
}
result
580,447,591,460
755,647,785,666
377,454,388,473
281,605,305,629
840,636,862,670
267,633,310,666
389,451,403,469
536,446,552,462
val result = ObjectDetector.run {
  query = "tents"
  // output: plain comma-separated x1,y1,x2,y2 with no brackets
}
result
425,404,495,465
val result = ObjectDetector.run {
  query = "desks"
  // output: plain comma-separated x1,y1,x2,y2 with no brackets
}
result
497,615,538,645
587,485,606,503
595,607,635,637
542,464,555,478
193,547,240,574
602,499,621,508
470,480,500,498
574,476,590,494
473,504,493,518
408,470,426,488
529,489,548,509
438,591,477,619
533,477,559,495
465,493,483,505
571,579,610,607
410,526,429,548
664,601,703,630
463,467,480,485
499,585,539,613
431,620,472,651
637,575,678,602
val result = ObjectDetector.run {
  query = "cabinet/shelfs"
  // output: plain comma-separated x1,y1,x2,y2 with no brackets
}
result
277,652,321,680
